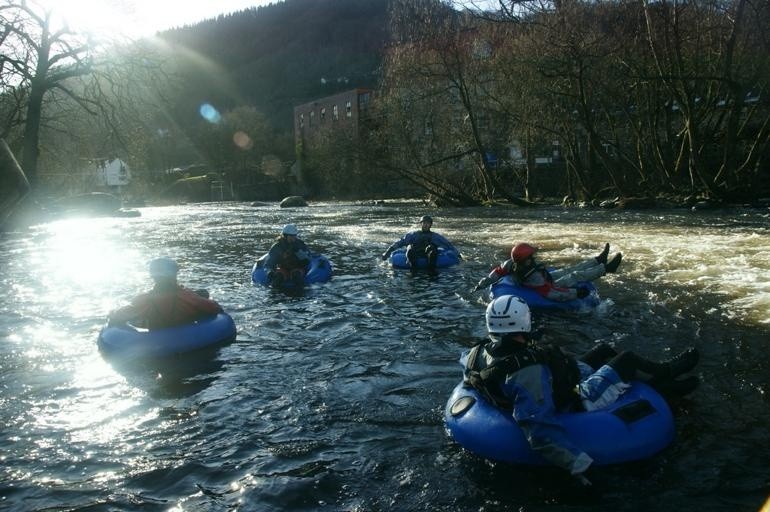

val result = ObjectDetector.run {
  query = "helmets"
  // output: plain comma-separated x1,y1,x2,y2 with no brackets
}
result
487,296,531,333
422,215,431,223
151,259,181,279
284,226,297,236
510,245,536,264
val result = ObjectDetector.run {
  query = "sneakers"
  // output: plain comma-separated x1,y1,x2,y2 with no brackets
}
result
608,254,622,274
669,348,699,380
663,375,695,400
596,245,611,265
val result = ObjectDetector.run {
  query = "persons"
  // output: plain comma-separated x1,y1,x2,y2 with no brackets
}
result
459,294,699,488
109,257,222,328
381,216,460,275
262,225,312,297
473,243,622,303
